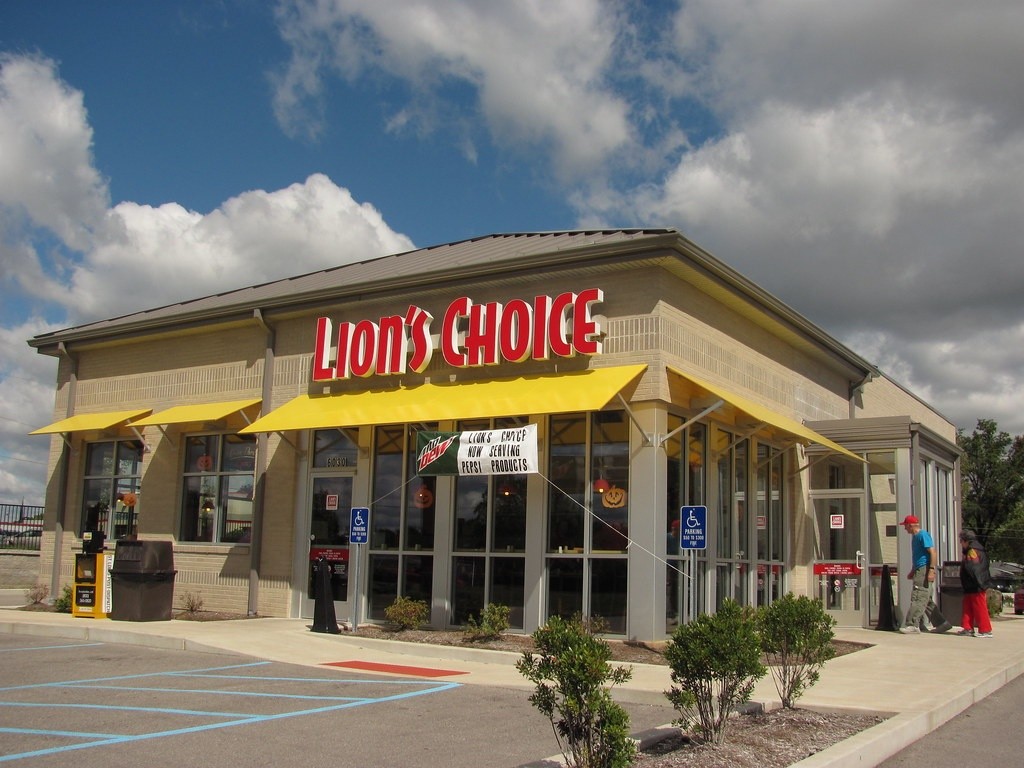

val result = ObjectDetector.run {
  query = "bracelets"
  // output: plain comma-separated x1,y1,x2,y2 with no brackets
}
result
929,566,935,570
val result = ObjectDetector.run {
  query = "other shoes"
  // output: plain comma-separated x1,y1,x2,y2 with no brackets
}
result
900,626,920,634
958,628,975,636
973,631,993,638
931,621,952,633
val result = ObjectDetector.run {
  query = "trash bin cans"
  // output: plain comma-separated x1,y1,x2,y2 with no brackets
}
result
107,539,178,623
940,561,962,626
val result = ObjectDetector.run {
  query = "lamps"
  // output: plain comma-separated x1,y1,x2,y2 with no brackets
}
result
202,477,215,511
116,480,125,502
497,475,518,495
594,417,609,492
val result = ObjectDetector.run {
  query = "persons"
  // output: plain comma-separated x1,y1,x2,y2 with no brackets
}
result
899,516,952,634
957,529,994,638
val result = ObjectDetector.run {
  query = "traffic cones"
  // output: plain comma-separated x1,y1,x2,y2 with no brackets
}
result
308,555,342,634
874,564,899,631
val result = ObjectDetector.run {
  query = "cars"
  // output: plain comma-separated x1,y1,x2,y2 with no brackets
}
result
1,529,42,550
989,564,1024,613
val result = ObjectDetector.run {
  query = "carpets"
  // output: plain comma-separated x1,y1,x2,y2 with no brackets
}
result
319,660,470,677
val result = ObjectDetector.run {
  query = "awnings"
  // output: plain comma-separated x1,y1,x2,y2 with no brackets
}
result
28,409,153,450
126,398,262,447
237,364,650,457
661,365,871,473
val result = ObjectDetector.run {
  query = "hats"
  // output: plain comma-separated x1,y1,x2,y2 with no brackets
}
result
960,529,975,541
900,516,919,525
